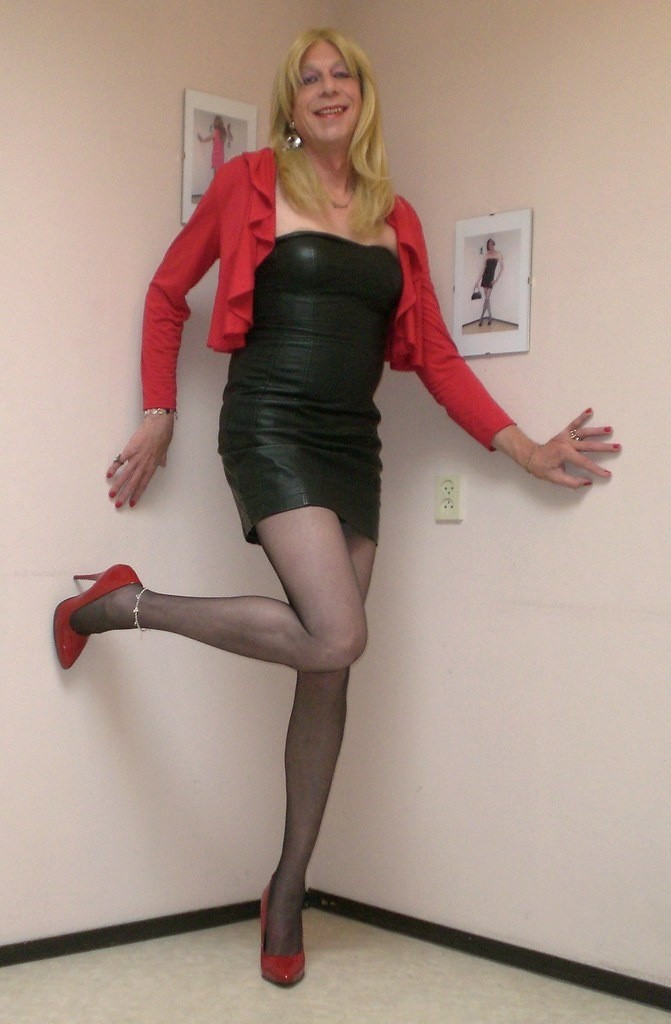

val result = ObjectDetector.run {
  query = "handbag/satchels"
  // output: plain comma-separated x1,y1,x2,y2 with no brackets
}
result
471,286,481,300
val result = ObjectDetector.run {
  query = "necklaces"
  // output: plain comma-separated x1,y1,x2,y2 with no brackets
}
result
330,185,356,208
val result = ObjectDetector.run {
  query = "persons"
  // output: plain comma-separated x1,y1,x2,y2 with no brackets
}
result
198,115,233,171
52,28,620,989
476,239,504,326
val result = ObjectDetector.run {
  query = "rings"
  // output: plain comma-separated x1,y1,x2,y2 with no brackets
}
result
113,454,124,465
570,429,583,441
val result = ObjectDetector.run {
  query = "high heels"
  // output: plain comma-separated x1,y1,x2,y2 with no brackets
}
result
259,875,305,984
54,564,141,669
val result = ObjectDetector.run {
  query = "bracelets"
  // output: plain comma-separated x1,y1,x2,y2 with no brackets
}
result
527,445,544,473
143,408,176,416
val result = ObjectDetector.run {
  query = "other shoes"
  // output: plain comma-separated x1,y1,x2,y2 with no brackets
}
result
487,319,492,325
479,319,483,327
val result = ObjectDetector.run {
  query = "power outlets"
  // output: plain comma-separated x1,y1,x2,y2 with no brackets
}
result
434,473,462,522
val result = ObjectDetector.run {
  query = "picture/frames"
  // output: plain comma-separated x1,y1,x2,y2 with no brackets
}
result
455,208,529,358
179,87,257,223
